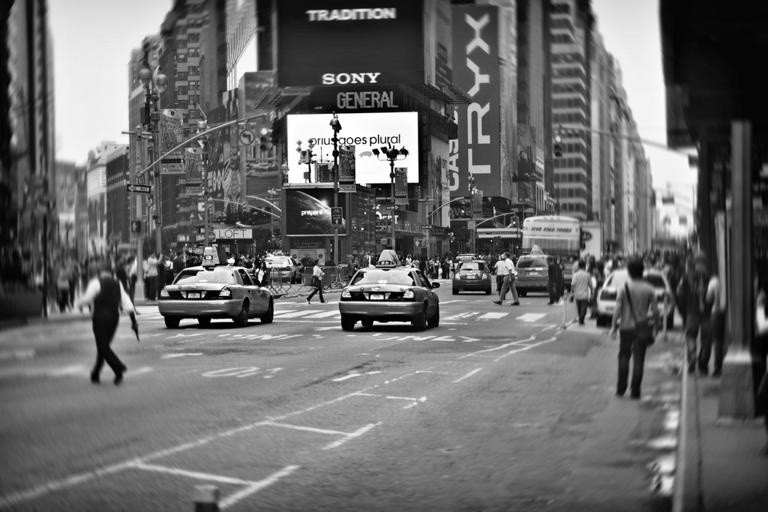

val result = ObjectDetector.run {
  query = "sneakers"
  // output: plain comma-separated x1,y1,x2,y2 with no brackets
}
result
92,365,127,385
618,381,640,398
579,318,584,324
687,354,721,377
494,301,519,305
549,298,559,304
306,298,328,304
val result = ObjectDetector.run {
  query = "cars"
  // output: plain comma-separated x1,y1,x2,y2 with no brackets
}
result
157,255,274,329
452,252,575,295
338,263,441,332
264,255,320,273
594,266,677,329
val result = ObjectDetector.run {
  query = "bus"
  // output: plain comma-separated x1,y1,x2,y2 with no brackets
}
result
521,213,584,293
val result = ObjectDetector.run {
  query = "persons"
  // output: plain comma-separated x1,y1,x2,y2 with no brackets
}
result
1,245,204,316
570,255,626,325
494,251,519,305
547,256,558,304
346,259,358,284
78,259,135,385
642,245,726,377
325,255,336,266
307,260,326,303
397,255,451,279
611,259,661,398
224,252,268,287
479,253,517,300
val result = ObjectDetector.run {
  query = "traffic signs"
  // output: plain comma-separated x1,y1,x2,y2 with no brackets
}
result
126,183,152,192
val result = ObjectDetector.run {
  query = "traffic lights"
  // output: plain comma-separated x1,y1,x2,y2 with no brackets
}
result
460,204,466,214
550,133,564,158
258,124,271,153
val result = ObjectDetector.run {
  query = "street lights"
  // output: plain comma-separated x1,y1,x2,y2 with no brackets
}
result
330,110,341,266
372,142,409,251
138,36,169,258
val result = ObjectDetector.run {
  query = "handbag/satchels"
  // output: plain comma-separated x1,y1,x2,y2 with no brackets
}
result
312,276,321,288
636,320,654,344
